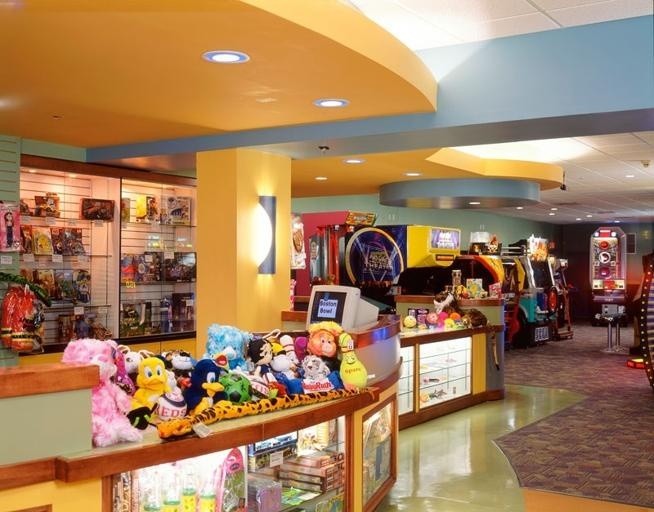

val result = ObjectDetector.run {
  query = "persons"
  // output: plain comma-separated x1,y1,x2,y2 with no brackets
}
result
309,239,320,279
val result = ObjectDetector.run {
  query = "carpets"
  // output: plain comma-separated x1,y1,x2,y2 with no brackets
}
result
491,322,654,508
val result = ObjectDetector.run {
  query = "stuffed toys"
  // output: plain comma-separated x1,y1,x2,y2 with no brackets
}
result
61,320,382,451
401,291,487,330
62,321,381,450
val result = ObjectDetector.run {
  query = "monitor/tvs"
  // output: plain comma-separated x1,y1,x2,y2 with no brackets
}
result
306,285,380,330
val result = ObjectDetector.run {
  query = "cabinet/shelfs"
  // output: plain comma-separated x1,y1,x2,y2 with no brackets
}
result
19,153,196,355
